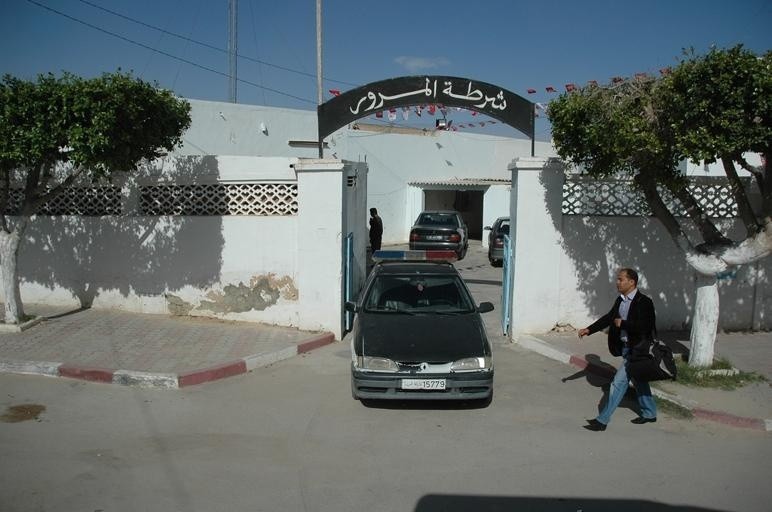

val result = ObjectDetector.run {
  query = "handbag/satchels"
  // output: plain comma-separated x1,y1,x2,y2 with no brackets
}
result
625,327,678,384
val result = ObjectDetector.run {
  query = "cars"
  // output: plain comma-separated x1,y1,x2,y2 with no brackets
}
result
409,210,469,259
483,217,510,267
345,249,495,403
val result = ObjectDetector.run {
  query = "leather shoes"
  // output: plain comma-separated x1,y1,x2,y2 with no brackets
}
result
584,418,608,431
630,415,658,425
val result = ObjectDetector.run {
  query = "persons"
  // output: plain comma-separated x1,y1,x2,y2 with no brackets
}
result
578,267,657,431
368,208,383,268
